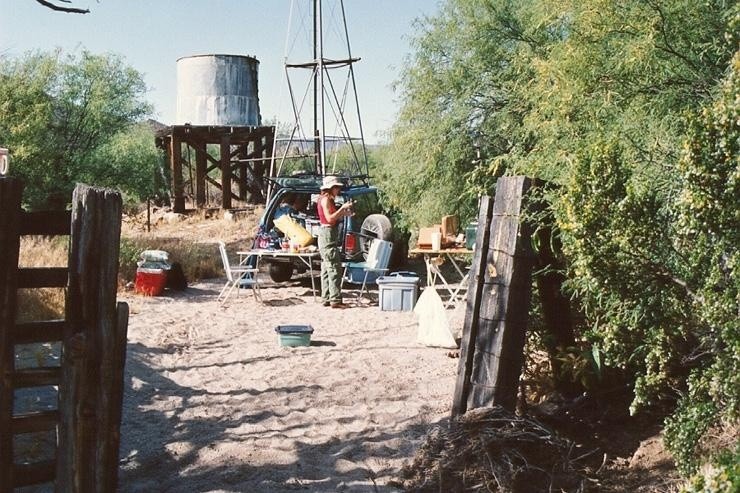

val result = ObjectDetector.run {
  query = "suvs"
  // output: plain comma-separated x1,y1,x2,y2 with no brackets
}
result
258,175,411,283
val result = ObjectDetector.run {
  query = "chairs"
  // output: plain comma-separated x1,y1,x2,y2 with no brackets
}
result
337,237,394,303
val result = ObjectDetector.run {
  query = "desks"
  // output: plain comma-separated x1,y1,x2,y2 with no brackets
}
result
235,249,320,302
409,247,474,310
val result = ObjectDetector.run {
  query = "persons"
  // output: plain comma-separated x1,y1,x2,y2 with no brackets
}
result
318,176,357,308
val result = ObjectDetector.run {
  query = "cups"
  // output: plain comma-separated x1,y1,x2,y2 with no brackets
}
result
431,232,442,250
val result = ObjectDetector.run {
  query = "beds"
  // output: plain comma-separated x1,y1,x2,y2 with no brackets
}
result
215,242,263,306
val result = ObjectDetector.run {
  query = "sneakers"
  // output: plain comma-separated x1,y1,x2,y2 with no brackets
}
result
324,303,349,308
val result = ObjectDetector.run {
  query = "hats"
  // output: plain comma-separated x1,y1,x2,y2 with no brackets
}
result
319,175,344,190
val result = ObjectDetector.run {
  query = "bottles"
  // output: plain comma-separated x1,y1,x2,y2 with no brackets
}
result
291,234,300,252
280,237,290,251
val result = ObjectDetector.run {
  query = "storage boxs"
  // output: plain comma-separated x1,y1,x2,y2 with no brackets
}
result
273,325,314,347
417,214,459,250
376,276,420,313
136,268,168,297
